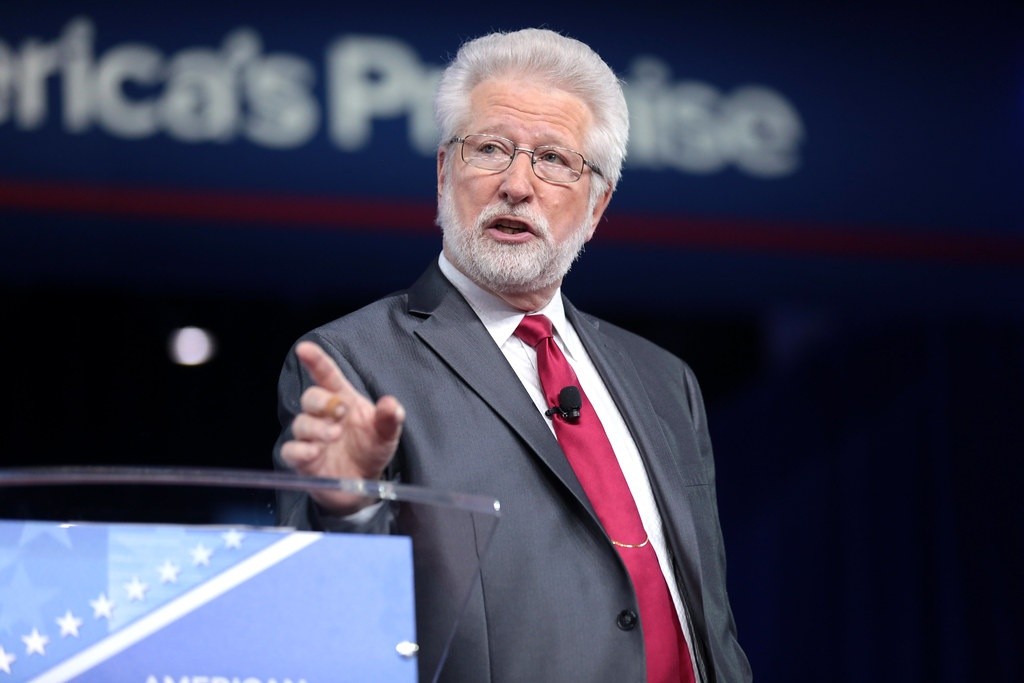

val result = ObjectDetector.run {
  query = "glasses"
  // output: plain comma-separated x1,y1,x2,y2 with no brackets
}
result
449,131,605,184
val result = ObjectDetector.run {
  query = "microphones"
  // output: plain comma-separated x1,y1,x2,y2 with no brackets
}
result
559,385,582,420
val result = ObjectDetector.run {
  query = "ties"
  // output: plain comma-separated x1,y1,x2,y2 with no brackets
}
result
510,312,698,683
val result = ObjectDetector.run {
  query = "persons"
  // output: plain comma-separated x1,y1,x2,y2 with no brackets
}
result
272,27,754,683
